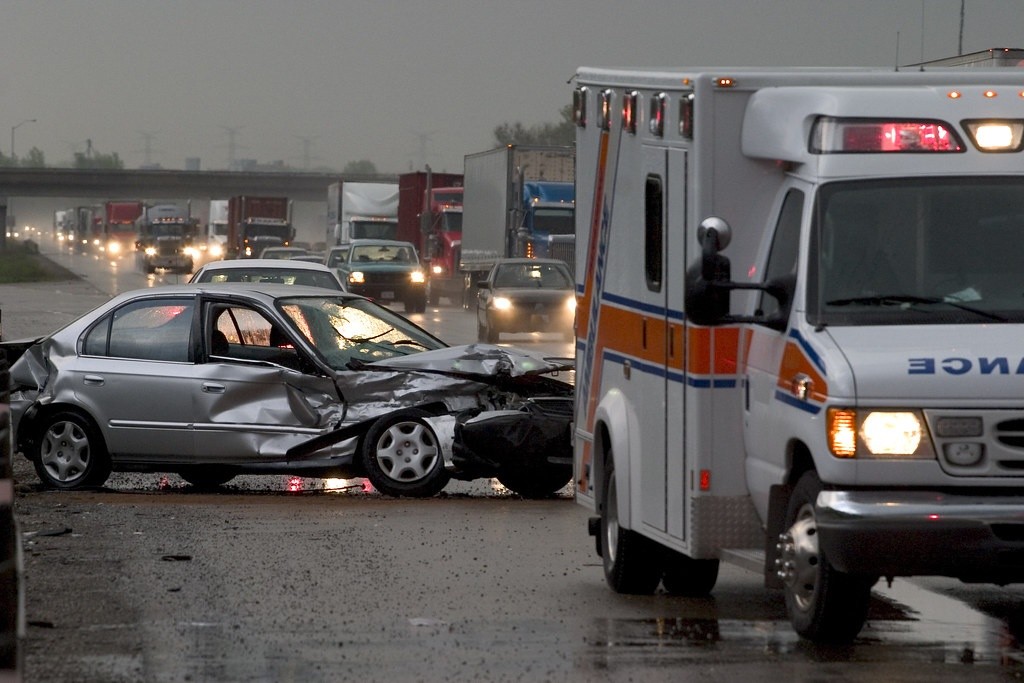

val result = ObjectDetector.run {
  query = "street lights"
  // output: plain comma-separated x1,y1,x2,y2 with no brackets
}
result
10,117,38,161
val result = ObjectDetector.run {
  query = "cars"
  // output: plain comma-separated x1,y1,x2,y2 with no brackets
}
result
0,279,580,499
475,258,577,343
257,246,352,268
337,237,429,315
186,258,348,296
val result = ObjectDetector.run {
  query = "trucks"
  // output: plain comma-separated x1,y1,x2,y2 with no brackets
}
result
559,56,1024,641
64,208,77,249
53,210,64,243
208,199,229,259
103,200,143,261
228,195,296,261
397,163,464,307
136,197,195,274
77,205,103,257
458,143,577,310
327,179,400,244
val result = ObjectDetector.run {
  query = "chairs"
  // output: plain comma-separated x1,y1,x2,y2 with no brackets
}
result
825,250,903,309
211,330,230,357
271,324,310,372
